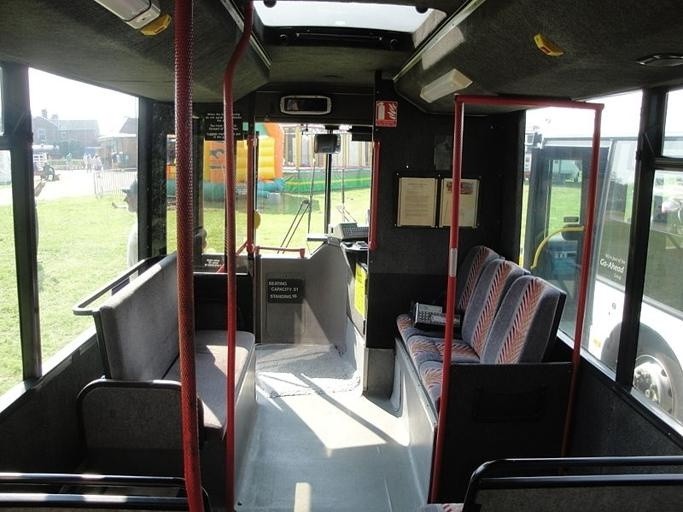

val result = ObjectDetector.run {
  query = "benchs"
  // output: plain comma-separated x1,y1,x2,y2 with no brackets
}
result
72,250,258,508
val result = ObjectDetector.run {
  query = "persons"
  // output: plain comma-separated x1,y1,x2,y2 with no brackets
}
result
93,155,102,178
110,153,118,170
41,162,55,182
119,178,138,273
123,151,130,167
64,153,73,171
85,155,92,172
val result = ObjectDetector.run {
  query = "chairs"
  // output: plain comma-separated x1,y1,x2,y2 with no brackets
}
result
396,244,506,340
407,256,530,368
417,275,570,409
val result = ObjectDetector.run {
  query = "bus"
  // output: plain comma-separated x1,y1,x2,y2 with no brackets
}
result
524,121,682,427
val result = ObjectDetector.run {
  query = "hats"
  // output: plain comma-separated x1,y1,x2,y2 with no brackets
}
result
121,181,137,194
